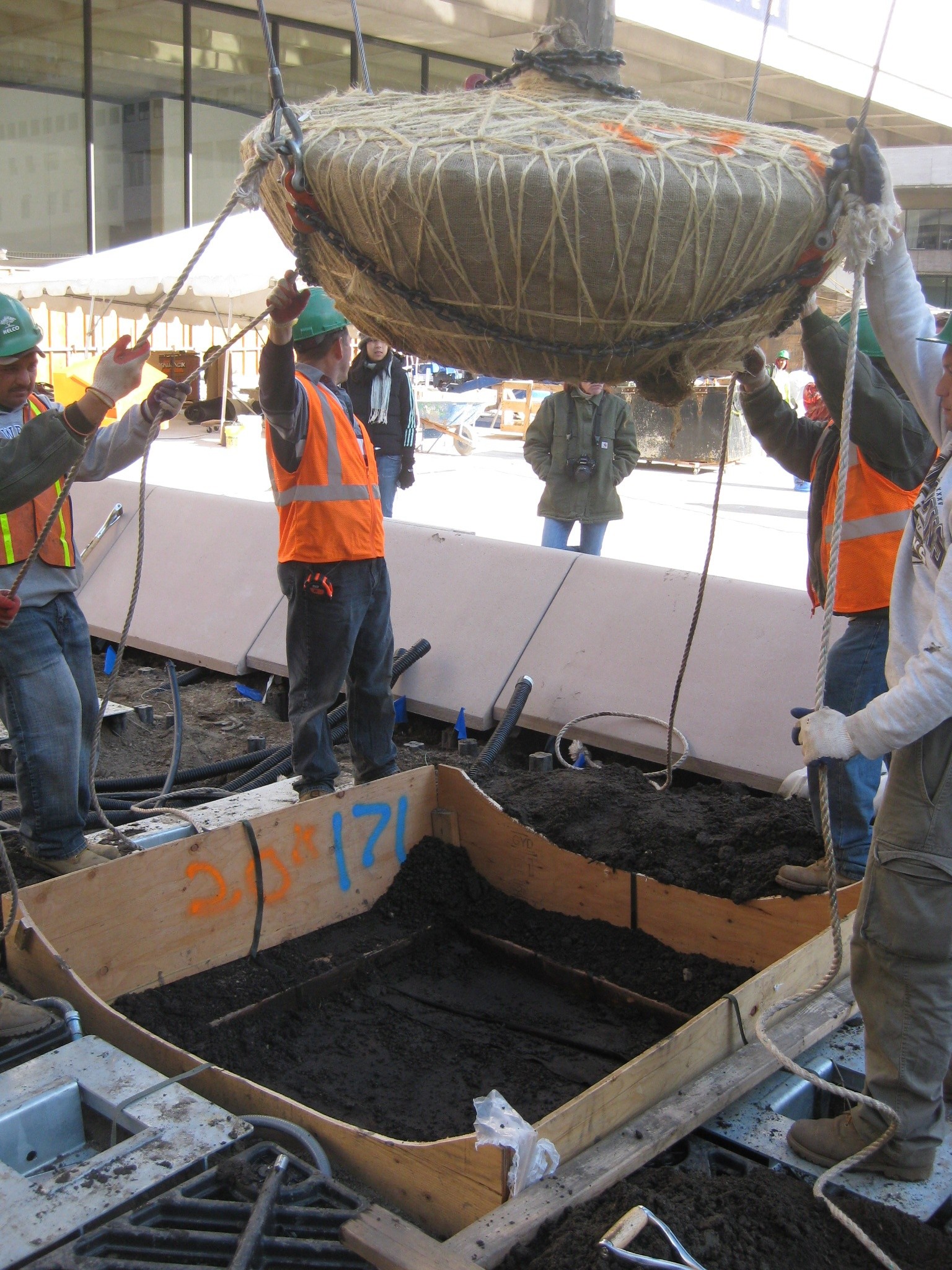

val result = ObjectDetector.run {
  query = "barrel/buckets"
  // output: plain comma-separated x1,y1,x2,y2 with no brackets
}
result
225,426,245,448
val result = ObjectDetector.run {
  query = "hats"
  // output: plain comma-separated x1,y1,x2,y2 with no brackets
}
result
0,345,46,366
358,332,375,349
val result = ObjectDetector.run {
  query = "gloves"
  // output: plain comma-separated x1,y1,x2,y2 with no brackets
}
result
87,335,150,409
806,292,817,307
141,378,192,427
266,270,311,329
397,458,415,490
824,116,904,224
790,706,852,769
728,341,766,386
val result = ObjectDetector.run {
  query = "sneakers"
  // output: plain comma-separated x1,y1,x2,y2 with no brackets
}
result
775,854,855,892
786,1108,935,1182
0,992,49,1037
299,790,331,804
86,844,119,860
25,843,112,875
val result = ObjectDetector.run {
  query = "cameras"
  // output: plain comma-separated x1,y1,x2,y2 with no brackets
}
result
566,454,596,483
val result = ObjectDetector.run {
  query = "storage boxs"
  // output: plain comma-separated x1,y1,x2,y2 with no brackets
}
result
159,355,200,404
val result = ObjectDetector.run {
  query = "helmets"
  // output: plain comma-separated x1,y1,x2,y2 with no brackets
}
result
837,308,886,357
777,350,789,359
292,285,352,342
0,294,43,358
915,311,952,344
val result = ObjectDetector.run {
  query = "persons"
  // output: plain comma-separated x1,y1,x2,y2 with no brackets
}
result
260,270,401,800
790,112,952,1181
0,289,192,871
523,382,640,556
338,329,417,516
734,299,934,884
765,350,831,492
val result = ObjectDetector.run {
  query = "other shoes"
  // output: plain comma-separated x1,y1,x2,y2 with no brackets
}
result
794,484,810,491
